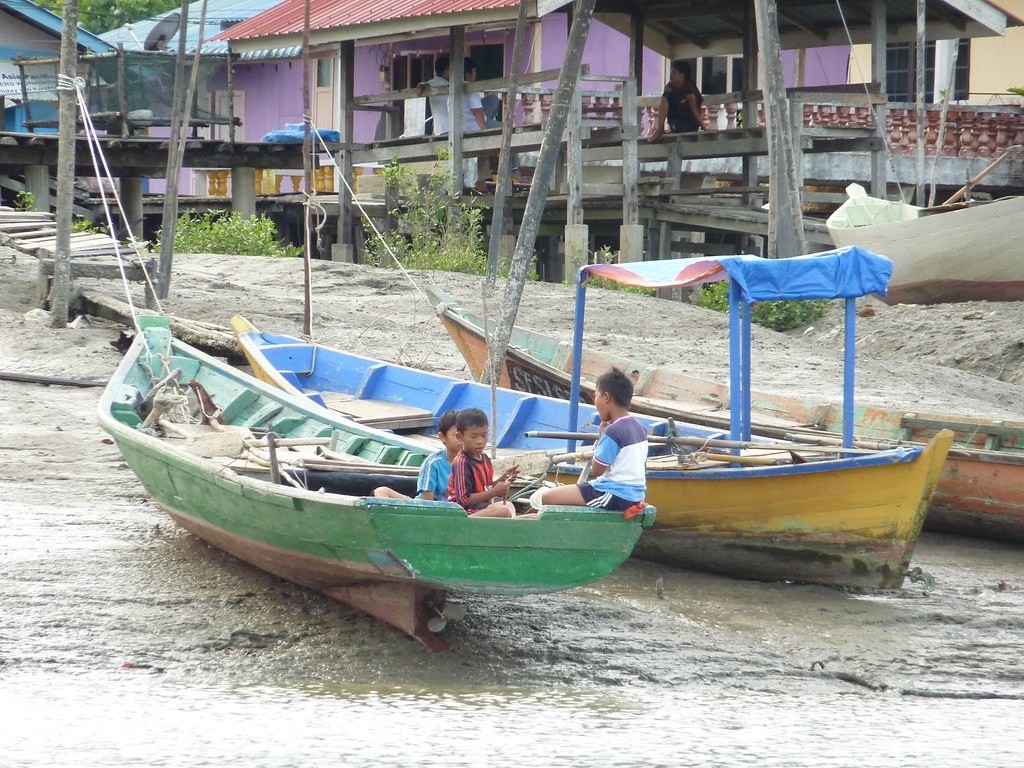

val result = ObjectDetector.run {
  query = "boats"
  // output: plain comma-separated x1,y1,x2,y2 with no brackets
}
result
824,180,1024,307
420,272,1024,543
92,310,658,658
229,244,956,592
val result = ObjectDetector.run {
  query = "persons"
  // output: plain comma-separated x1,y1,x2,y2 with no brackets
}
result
648,61,716,141
541,366,649,510
374,410,464,502
447,407,521,518
415,55,525,195
